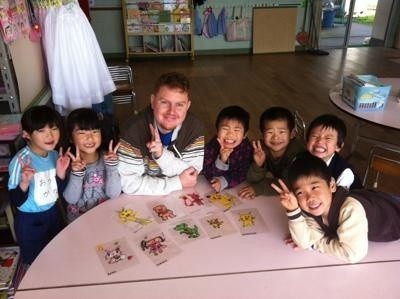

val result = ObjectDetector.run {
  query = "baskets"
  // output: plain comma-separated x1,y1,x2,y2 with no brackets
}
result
343,75,391,111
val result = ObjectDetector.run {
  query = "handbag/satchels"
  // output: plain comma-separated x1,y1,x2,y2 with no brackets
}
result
226,18,251,42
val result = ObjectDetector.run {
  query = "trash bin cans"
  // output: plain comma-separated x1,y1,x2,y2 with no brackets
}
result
323,9,335,28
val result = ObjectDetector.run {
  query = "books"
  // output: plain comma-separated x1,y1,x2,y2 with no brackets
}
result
0,245,30,299
349,73,385,88
128,38,189,53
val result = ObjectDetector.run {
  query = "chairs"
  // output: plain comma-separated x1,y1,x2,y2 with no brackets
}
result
361,145,400,187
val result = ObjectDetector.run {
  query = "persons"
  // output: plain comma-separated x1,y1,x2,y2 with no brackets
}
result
305,114,363,192
238,105,307,200
270,154,400,264
117,71,207,196
8,104,72,266
203,105,253,194
61,107,121,225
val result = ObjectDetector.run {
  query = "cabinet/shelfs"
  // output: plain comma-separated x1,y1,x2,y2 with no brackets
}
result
122,0,194,63
0,1,53,174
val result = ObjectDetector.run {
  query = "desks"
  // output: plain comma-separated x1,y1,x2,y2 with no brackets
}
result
328,78,400,188
13,176,400,299
